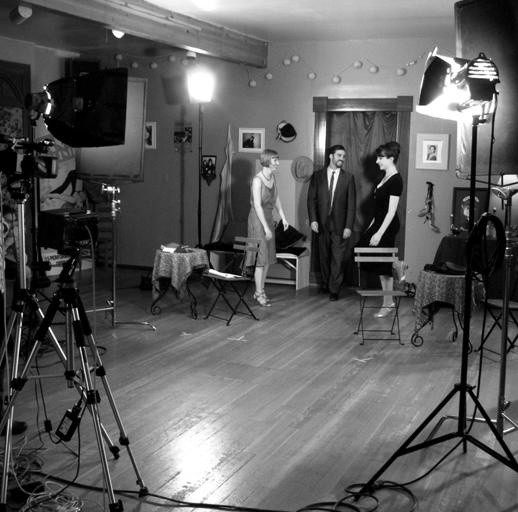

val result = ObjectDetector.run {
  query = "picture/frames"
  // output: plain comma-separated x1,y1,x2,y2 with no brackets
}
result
413,132,450,171
237,127,266,153
452,187,488,231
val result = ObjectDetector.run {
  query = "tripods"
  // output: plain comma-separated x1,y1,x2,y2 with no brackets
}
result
0,193,122,460
354,123,518,504
0,257,148,512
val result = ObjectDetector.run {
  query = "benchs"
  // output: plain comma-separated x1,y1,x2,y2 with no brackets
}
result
265,246,310,291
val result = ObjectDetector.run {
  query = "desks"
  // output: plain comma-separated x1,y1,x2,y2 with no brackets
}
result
409,267,485,354
433,235,500,291
150,247,206,320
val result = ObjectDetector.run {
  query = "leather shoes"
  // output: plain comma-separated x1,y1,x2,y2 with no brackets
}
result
329,294,337,301
319,288,327,294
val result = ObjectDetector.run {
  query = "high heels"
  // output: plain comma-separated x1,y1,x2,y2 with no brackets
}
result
375,303,395,318
253,288,272,307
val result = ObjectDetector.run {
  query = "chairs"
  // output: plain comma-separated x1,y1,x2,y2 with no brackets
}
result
200,237,262,326
353,246,407,346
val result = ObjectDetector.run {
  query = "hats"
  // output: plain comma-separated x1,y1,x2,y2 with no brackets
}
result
291,156,314,183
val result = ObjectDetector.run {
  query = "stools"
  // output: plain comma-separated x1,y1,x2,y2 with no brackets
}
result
473,297,517,358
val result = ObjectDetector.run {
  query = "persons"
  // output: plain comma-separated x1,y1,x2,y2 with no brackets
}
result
364,142,402,317
240,149,288,307
306,143,357,301
426,146,436,161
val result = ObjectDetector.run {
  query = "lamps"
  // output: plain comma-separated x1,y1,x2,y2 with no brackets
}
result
355,48,517,509
0,66,151,510
184,69,215,250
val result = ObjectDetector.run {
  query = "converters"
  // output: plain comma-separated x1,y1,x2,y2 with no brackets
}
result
55,409,81,442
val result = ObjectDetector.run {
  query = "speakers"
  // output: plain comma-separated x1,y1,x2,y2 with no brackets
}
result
455,0,518,177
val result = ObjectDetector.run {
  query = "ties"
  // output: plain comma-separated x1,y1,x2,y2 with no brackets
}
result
328,172,335,215
